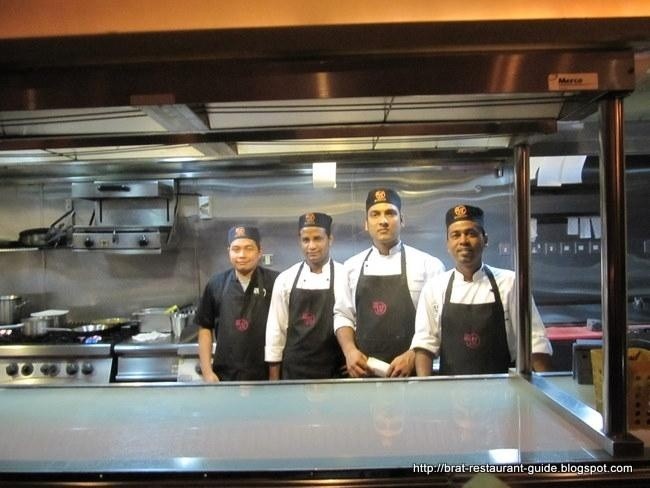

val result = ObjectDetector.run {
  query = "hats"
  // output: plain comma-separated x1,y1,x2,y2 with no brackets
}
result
445,205,483,225
227,225,260,245
299,212,333,230
366,188,401,209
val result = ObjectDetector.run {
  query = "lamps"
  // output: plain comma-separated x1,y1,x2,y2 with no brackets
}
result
313,162,337,189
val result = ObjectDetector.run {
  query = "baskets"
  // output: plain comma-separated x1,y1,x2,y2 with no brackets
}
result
591,348,650,429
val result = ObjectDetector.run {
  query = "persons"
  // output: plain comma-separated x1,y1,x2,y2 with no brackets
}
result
409,204,554,377
264,212,350,381
332,187,447,377
194,223,280,382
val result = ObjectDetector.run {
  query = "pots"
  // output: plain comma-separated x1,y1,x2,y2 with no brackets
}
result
0,295,129,338
19,209,74,248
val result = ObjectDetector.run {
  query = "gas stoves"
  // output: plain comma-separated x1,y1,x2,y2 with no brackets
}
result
0,322,139,386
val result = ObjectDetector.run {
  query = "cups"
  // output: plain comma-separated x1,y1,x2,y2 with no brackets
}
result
171,307,198,339
